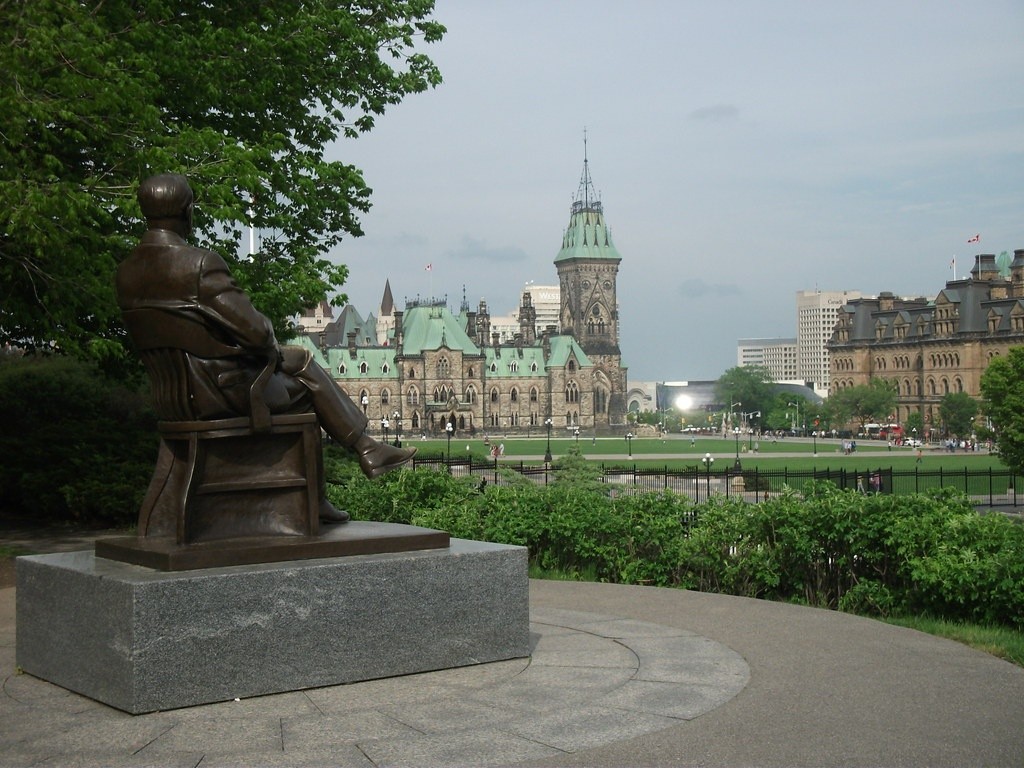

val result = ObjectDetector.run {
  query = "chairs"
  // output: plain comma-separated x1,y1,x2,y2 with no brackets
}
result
118,307,323,545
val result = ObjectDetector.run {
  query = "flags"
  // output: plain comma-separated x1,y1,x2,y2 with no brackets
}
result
425,264,432,271
967,233,979,243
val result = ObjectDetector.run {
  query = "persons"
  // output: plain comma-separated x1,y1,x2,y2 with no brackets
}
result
489,441,506,458
888,440,892,452
916,450,922,464
755,428,785,441
421,433,427,441
691,436,695,447
840,438,858,455
113,172,419,523
941,437,991,453
754,442,758,454
741,443,747,455
856,472,881,496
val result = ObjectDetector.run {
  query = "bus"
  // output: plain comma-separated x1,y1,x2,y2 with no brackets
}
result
858,423,904,440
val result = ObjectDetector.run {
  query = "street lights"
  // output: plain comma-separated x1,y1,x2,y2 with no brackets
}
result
911,428,917,450
734,410,762,439
702,453,714,500
392,411,400,448
730,402,742,430
732,426,743,458
503,422,507,438
526,421,532,438
362,395,370,418
930,428,935,445
574,429,580,446
627,432,632,457
747,428,754,450
812,431,817,455
380,420,386,444
543,418,554,464
788,393,799,428
383,420,389,445
445,422,454,474
658,421,663,438
